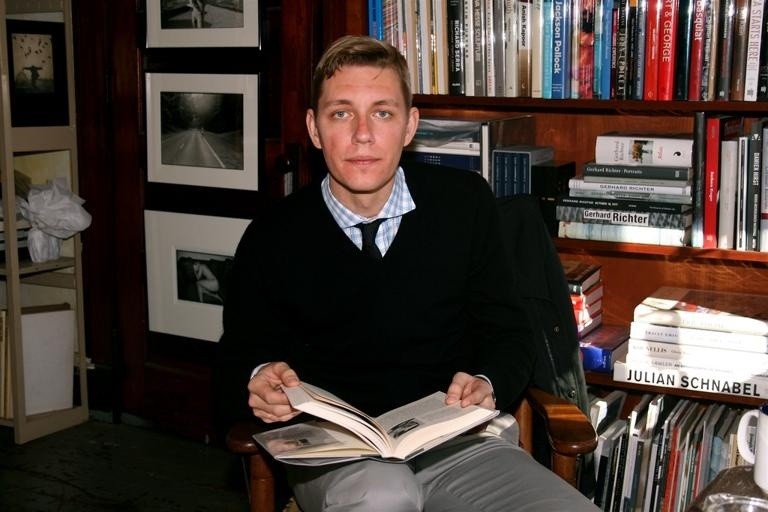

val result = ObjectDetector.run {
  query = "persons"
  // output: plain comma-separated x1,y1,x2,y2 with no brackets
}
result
23,65,42,87
190,0,205,28
223,35,603,511
183,259,224,303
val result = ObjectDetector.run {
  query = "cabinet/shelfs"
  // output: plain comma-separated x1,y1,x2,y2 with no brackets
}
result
296,1,768,432
0,0,93,450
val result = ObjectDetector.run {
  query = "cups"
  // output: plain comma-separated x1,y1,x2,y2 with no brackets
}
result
735,403,768,491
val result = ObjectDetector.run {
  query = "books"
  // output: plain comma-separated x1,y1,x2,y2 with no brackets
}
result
406,109,768,252
369,0,768,101
252,379,501,467
559,259,768,511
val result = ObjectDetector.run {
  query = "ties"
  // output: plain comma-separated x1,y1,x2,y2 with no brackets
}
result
355,220,384,265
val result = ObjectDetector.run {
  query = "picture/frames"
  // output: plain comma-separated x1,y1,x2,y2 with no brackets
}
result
144,70,261,194
142,208,255,342
139,0,265,55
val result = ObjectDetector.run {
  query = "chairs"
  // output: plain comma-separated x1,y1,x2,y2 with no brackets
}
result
203,155,601,510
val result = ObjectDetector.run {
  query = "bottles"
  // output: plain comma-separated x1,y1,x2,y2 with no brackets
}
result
277,141,296,199
